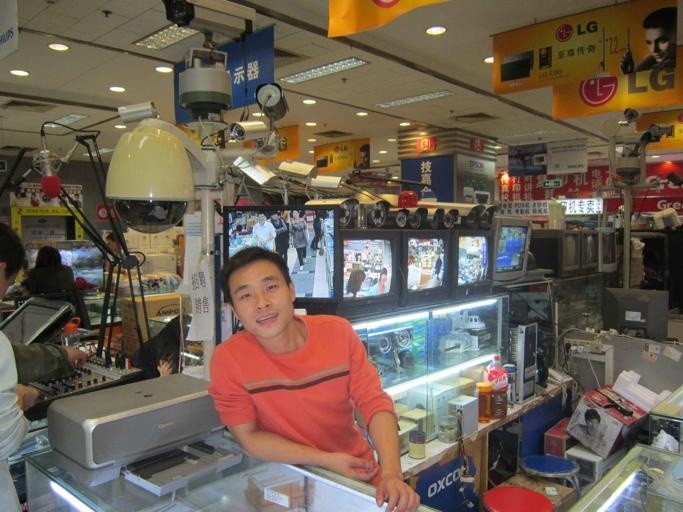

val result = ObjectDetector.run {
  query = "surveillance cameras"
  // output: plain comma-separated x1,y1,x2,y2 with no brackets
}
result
229,81,342,190
368,325,412,354
305,197,498,230
118,101,156,122
624,108,683,186
104,128,194,234
162,0,255,41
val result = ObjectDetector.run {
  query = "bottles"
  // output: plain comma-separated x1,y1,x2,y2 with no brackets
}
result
61,317,80,349
474,379,493,424
409,431,427,460
484,354,507,419
435,414,459,443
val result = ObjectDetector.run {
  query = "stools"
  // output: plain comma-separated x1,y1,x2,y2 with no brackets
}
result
483,451,582,511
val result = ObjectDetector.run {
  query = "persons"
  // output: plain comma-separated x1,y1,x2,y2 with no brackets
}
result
319,211,329,250
21,242,93,340
309,209,331,251
102,230,128,274
249,210,274,251
288,209,309,274
432,252,445,282
0,219,43,512
8,335,93,382
375,267,387,297
345,252,366,297
571,409,613,457
616,5,676,76
206,245,424,512
353,144,370,169
266,211,288,266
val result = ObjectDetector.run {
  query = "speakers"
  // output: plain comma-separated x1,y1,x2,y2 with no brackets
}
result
48,374,227,470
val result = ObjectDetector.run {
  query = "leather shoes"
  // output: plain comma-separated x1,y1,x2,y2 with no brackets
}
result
310,245,319,250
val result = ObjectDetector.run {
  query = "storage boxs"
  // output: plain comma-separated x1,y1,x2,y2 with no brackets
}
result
118,292,192,358
542,383,649,482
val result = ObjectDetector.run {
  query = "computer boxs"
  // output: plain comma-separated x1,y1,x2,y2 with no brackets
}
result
509,322,537,403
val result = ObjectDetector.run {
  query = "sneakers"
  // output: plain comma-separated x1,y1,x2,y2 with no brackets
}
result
302,258,307,263
299,266,304,271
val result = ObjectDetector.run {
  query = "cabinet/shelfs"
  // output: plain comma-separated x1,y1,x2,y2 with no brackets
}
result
352,381,574,512
567,443,683,512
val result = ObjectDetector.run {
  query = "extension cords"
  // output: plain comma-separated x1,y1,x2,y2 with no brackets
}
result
564,339,601,354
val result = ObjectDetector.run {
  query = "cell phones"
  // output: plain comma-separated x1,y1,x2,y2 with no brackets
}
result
539,47,552,69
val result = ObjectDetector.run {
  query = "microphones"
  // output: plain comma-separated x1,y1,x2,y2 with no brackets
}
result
40,131,61,198
14,168,32,185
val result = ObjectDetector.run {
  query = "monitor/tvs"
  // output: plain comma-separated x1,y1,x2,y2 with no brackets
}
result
0,297,72,348
501,50,533,88
603,288,669,340
341,220,617,316
224,206,340,313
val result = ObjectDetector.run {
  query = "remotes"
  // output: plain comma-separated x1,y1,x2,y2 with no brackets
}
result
593,387,633,416
